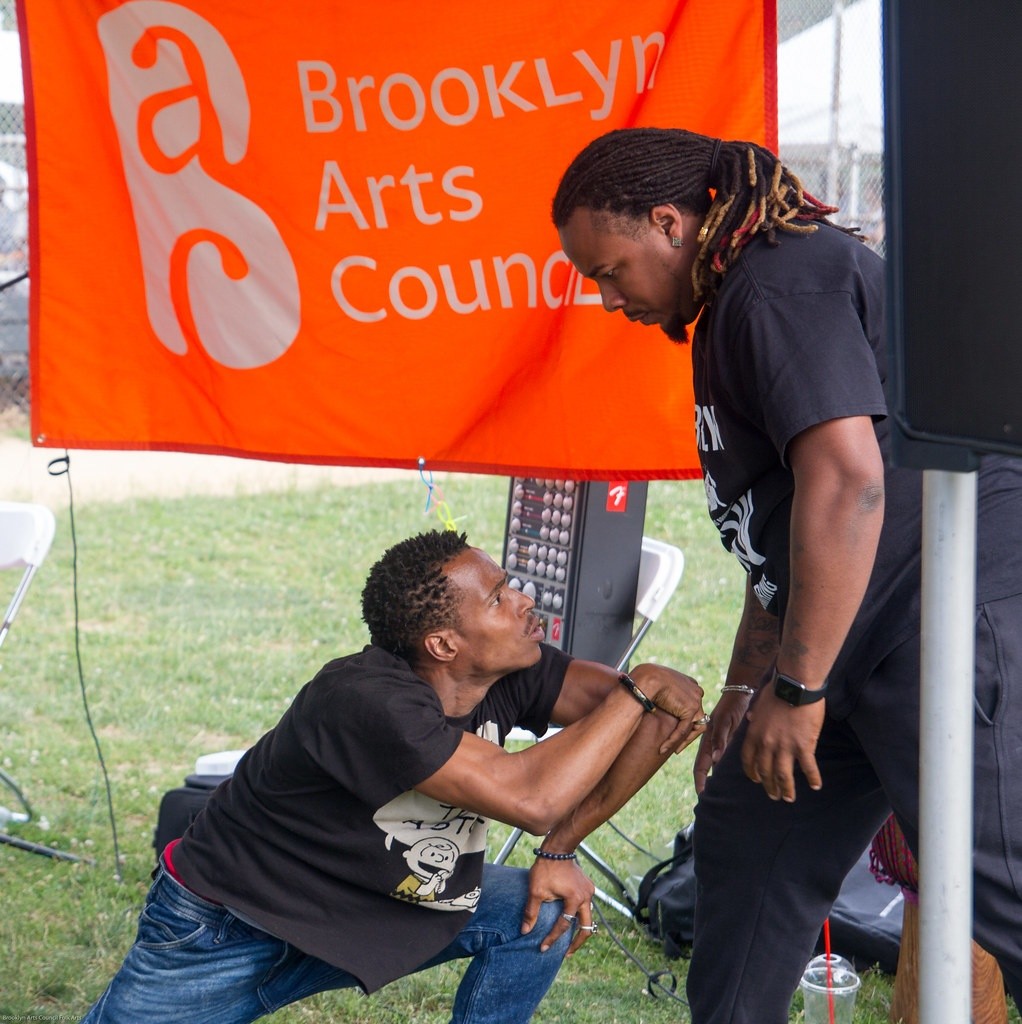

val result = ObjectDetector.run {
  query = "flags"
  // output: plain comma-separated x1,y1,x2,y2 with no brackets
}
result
15,0,779,483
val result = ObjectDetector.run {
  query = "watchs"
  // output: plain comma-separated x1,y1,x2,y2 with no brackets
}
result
771,667,830,706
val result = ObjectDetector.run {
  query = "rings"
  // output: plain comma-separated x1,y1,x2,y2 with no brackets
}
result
579,922,598,933
694,713,711,725
561,913,576,923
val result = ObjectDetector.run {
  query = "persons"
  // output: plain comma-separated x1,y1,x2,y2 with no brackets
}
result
551,125,1022,1024
77,530,710,1024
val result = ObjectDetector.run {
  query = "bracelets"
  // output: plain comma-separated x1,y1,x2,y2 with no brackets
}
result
721,685,761,694
618,672,656,714
533,848,576,859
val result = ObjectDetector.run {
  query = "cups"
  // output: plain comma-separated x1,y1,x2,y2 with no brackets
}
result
800,953,862,1024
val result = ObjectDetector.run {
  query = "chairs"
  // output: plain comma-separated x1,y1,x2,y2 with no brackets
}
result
480,532,688,923
0,497,58,834
